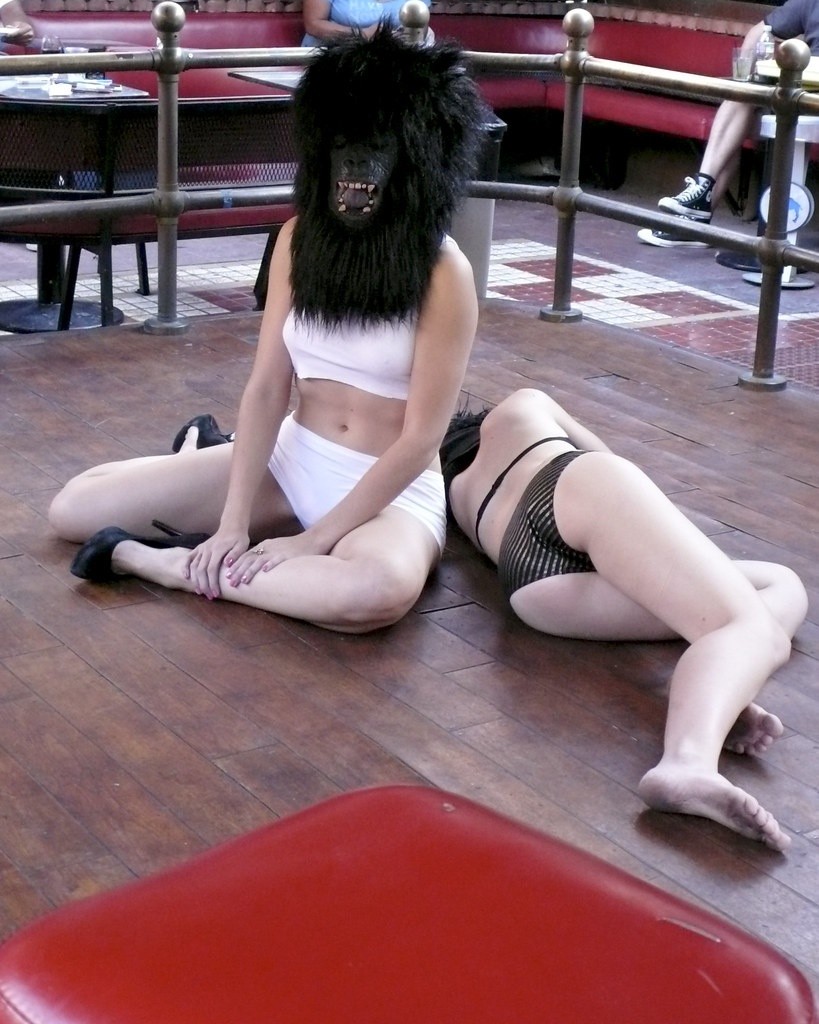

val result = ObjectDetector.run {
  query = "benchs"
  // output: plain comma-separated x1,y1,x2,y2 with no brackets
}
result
0,93,303,331
0,2,819,214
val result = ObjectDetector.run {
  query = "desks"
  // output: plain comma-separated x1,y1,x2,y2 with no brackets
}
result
716,74,819,292
227,70,308,312
0,76,150,334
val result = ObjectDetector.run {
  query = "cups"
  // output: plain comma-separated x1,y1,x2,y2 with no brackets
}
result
732,47,754,81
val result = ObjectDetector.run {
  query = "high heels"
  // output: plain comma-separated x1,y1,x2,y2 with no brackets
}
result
69,520,213,584
172,415,233,453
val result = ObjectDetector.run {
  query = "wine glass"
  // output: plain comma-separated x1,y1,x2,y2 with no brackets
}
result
42,37,64,91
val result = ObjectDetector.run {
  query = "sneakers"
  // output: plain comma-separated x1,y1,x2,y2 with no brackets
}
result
636,221,710,249
658,173,712,219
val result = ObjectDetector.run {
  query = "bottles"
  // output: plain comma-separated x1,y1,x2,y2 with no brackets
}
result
753,25,775,82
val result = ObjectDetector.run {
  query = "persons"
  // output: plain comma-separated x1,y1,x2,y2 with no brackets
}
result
637,0,819,250
439,387,808,851
48,12,488,633
300,0,432,47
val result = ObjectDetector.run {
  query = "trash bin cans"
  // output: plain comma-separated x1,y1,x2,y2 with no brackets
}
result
443,99,508,308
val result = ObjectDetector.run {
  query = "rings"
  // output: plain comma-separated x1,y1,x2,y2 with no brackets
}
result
256,548,263,555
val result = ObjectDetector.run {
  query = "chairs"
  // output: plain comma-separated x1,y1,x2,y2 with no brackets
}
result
0,783,819,1024
